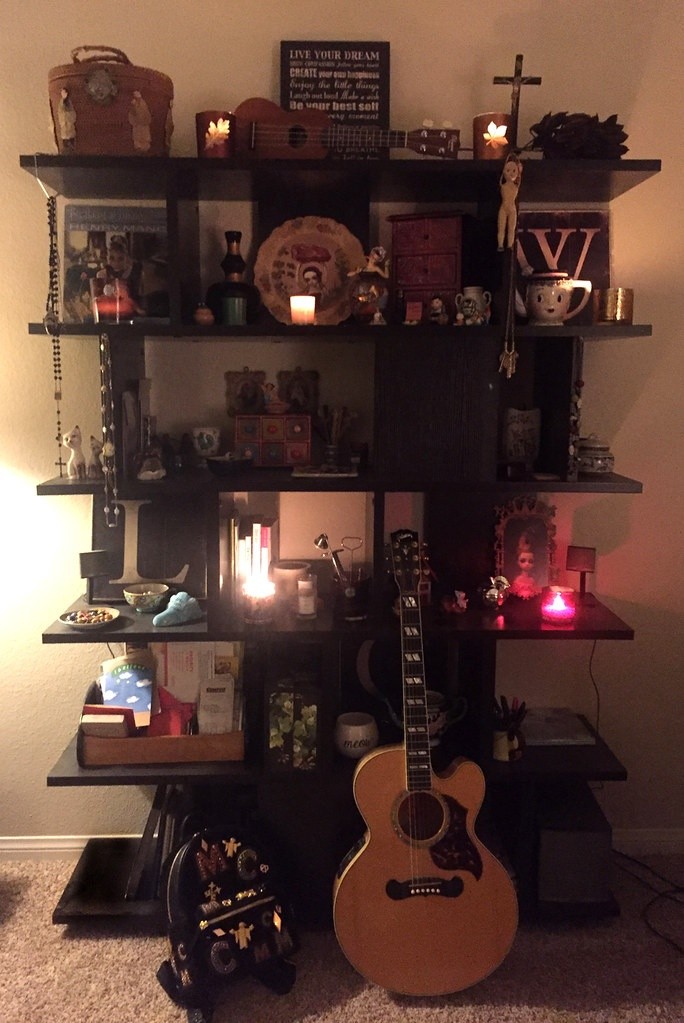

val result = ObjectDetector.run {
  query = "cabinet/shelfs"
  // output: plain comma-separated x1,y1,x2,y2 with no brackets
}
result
386,208,475,323
20,155,661,922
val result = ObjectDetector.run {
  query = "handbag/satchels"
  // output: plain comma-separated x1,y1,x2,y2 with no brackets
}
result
156,814,299,1006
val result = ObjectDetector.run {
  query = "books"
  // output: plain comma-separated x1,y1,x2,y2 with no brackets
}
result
230,509,279,610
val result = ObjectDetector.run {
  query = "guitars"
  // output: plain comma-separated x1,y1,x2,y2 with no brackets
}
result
332,528,520,999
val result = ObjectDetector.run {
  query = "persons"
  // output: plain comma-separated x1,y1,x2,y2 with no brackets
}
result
497,161,523,253
346,246,390,312
430,296,443,321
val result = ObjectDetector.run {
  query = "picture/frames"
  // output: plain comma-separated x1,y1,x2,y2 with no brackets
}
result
88,489,209,603
282,39,390,156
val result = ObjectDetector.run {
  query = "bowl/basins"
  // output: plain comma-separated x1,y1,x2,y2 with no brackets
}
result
123,583,170,613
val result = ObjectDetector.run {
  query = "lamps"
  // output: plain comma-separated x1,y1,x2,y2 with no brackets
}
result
242,580,274,627
538,586,575,623
290,296,315,324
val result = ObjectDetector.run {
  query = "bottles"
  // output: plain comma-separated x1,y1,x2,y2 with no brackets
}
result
293,575,318,620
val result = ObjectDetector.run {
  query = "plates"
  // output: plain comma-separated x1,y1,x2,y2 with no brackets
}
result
58,608,120,630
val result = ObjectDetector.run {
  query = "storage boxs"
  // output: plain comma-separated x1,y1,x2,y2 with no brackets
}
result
74,681,245,764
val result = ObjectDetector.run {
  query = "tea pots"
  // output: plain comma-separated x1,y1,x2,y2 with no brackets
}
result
573,434,615,477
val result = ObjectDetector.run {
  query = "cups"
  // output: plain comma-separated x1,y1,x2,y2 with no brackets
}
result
196,110,237,158
334,713,380,759
243,592,273,625
490,730,519,762
331,571,372,622
472,113,514,160
272,562,317,603
